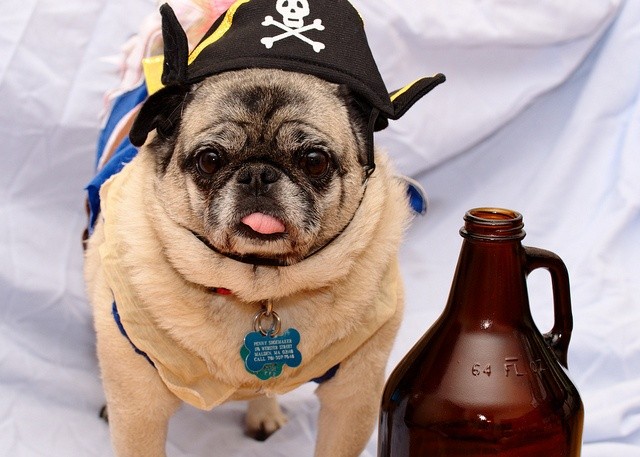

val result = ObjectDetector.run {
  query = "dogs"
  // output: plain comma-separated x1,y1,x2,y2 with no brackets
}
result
75,66,416,457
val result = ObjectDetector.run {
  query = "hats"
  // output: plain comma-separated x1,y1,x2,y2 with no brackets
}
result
161,0,446,119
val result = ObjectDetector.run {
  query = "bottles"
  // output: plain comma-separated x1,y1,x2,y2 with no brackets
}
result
375,205,585,457
